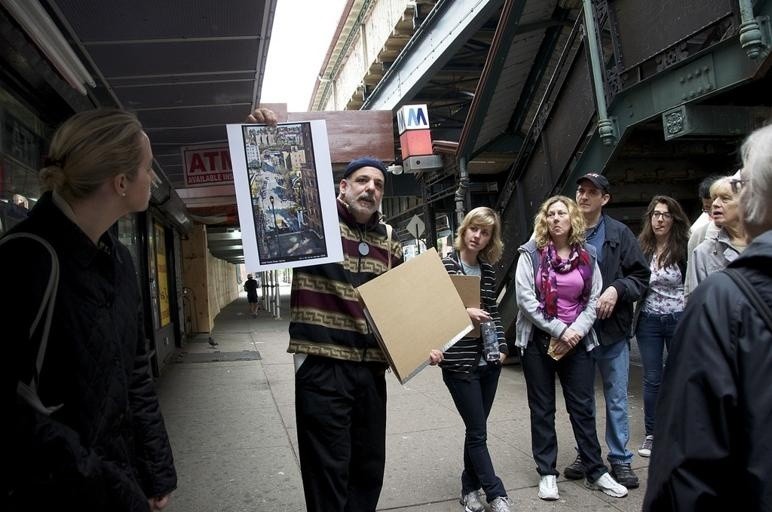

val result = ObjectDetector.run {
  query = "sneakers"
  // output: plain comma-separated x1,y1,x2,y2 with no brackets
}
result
584,472,629,498
637,435,656,457
564,448,590,480
537,475,560,500
610,462,640,489
460,490,485,512
491,496,510,512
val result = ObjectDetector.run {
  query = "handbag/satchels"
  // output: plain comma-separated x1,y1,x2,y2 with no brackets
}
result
7,233,63,500
721,264,770,338
243,284,248,291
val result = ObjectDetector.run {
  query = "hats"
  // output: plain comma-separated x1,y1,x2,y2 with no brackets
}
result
247,274,253,279
343,157,390,190
576,173,610,192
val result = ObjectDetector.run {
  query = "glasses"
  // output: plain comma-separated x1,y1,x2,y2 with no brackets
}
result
702,205,710,212
575,188,599,197
650,211,673,219
730,180,745,194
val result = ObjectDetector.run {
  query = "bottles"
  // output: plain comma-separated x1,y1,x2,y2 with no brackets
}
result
481,314,501,362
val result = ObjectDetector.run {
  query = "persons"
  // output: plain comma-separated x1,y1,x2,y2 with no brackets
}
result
631,194,691,459
245,105,443,512
689,176,748,299
244,274,262,317
514,195,629,502
435,207,510,512
641,122,772,512
564,173,651,490
0,108,179,512
683,175,728,313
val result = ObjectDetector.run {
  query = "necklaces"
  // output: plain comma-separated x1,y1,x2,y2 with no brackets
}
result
356,221,371,256
